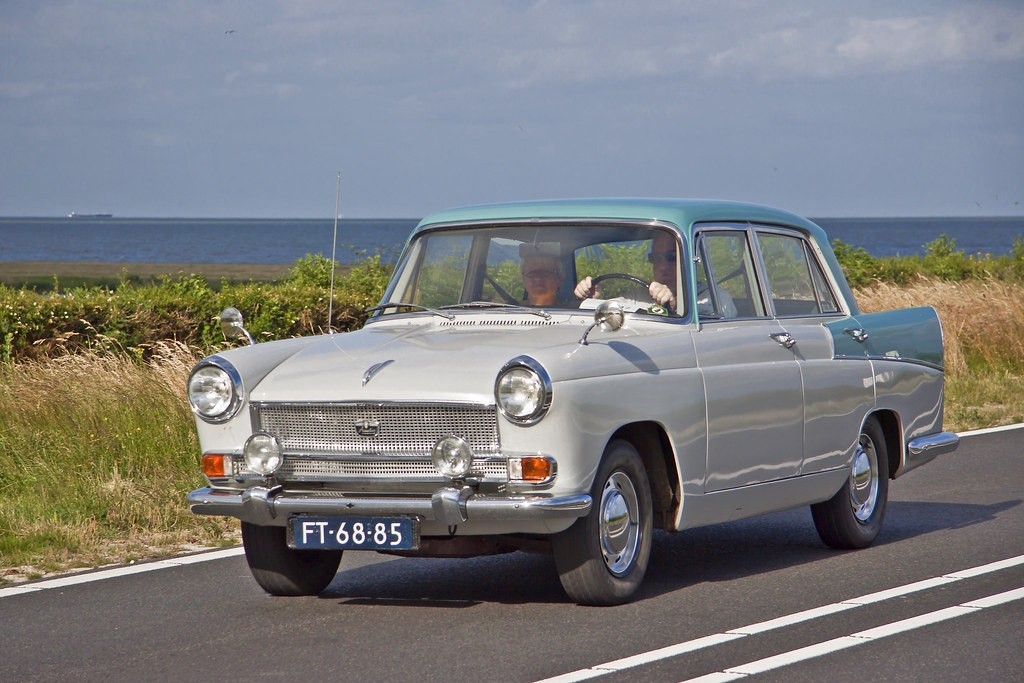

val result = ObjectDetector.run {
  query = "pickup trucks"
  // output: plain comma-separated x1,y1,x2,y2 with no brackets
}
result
187,198,962,608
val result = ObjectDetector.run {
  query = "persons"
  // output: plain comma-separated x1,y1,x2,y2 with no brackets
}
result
574,233,737,318
518,250,564,306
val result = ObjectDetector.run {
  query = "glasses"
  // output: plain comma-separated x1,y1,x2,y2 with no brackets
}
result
523,268,558,279
648,250,676,263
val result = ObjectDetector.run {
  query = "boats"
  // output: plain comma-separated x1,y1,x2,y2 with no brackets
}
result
69,212,112,217
